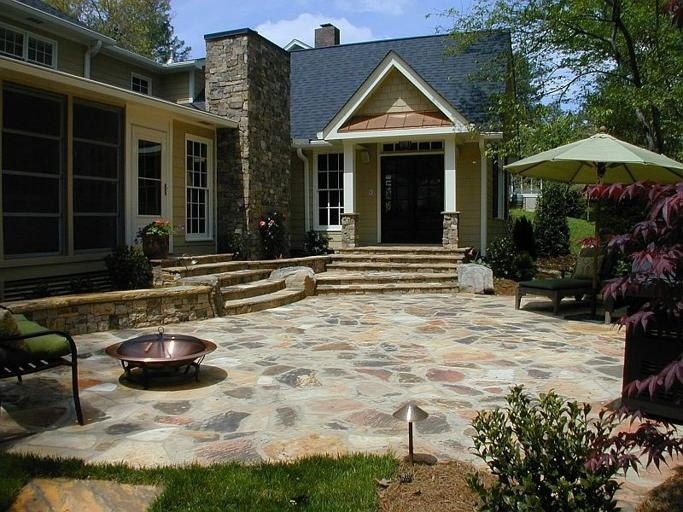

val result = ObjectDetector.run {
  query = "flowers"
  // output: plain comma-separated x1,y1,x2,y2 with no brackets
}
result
137,216,180,237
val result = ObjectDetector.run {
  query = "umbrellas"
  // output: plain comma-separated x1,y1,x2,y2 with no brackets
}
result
501,124,683,321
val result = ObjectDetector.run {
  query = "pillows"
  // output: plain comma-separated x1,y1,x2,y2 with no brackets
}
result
0,303,31,359
571,251,604,279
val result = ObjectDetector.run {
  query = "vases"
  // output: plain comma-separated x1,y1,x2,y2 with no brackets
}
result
140,233,171,259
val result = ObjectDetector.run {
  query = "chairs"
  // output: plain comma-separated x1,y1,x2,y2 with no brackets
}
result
511,245,618,316
0,301,89,428
